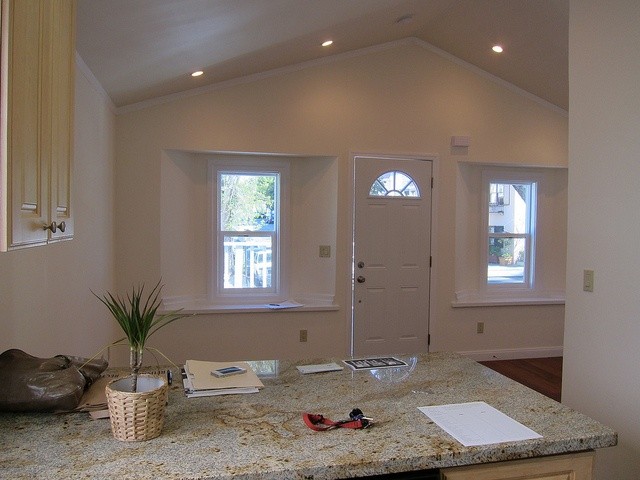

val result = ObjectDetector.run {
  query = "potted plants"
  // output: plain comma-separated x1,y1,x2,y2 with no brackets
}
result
74,275,189,443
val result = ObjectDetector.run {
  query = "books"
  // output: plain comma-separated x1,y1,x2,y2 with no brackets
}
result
181,359,265,399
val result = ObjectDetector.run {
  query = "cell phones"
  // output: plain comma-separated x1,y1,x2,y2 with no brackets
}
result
211,366,248,379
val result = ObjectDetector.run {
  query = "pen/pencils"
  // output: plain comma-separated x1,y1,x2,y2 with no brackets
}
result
168,369,172,384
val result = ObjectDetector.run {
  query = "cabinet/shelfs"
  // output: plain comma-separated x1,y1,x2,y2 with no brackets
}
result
440,448,594,479
0,0,79,253
